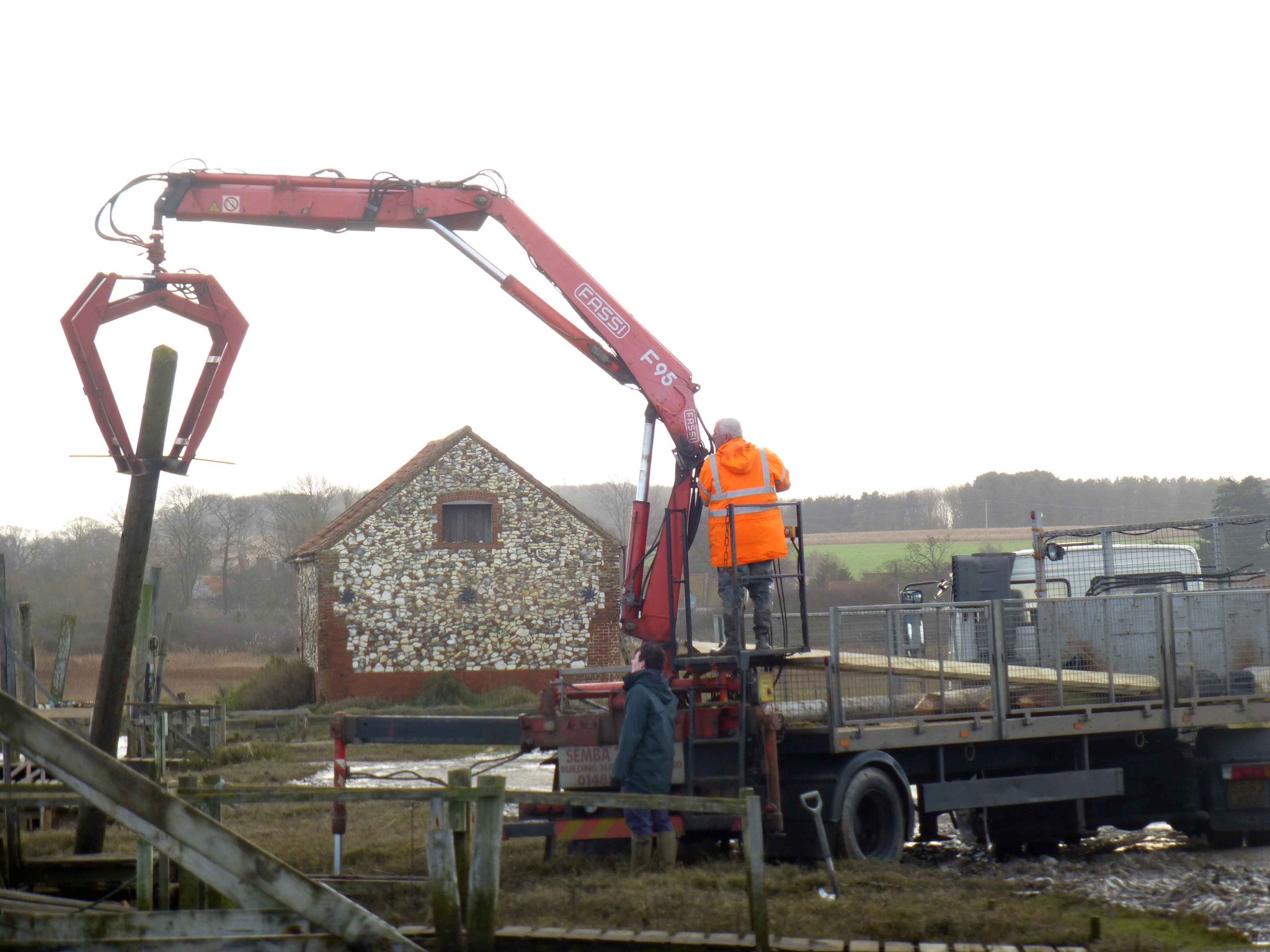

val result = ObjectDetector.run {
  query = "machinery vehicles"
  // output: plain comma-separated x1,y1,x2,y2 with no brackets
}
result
59,157,810,901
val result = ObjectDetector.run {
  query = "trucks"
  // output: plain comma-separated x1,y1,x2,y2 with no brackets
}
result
901,543,1270,862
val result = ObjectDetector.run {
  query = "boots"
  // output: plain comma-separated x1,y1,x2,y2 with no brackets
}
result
710,607,746,656
629,836,653,877
654,832,677,872
751,609,772,649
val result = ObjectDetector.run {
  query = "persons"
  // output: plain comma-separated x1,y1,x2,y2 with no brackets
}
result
607,643,677,879
697,418,790,656
